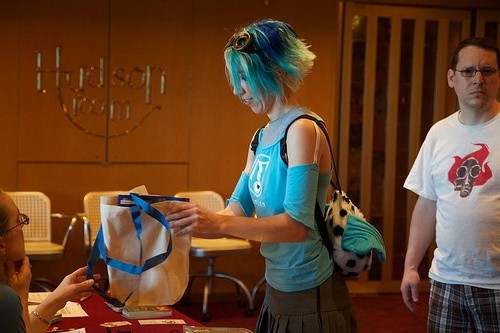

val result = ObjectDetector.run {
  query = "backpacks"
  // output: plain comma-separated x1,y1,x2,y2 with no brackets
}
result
251,114,386,277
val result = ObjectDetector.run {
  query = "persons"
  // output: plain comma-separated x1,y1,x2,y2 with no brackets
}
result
0,189,101,333
166,18,358,333
400,36,500,333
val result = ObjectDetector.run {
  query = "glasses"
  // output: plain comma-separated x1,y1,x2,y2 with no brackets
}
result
223,32,288,75
0,213,29,235
455,67,499,77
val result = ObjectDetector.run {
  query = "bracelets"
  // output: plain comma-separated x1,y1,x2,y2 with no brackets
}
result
32,311,51,327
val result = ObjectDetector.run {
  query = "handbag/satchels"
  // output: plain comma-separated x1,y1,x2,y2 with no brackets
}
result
87,192,192,307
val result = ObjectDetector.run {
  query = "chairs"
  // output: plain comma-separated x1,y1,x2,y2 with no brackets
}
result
78,191,128,261
170,190,255,322
4,191,78,292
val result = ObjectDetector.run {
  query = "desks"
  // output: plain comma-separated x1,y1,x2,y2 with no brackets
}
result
43,289,206,333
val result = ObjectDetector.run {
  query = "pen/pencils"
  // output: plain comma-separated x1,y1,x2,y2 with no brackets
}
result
80,295,91,301
